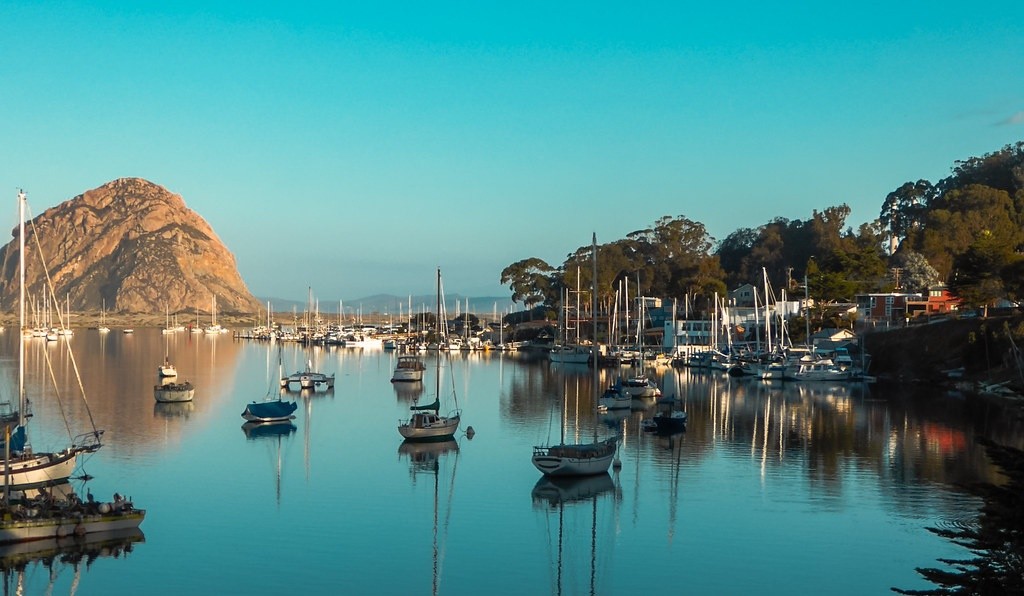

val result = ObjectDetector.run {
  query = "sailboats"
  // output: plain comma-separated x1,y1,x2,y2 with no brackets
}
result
247,294,529,351
548,263,879,434
22,284,73,341
532,472,616,596
161,293,230,335
157,304,177,377
99,298,111,332
280,287,335,389
531,232,624,478
397,436,461,595
396,266,463,439
0,185,106,491
241,419,298,509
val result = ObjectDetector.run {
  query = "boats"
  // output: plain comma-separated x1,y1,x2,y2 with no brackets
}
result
0,492,146,545
0,397,35,447
0,527,146,575
153,380,195,403
391,356,427,381
123,327,134,333
241,333,300,421
